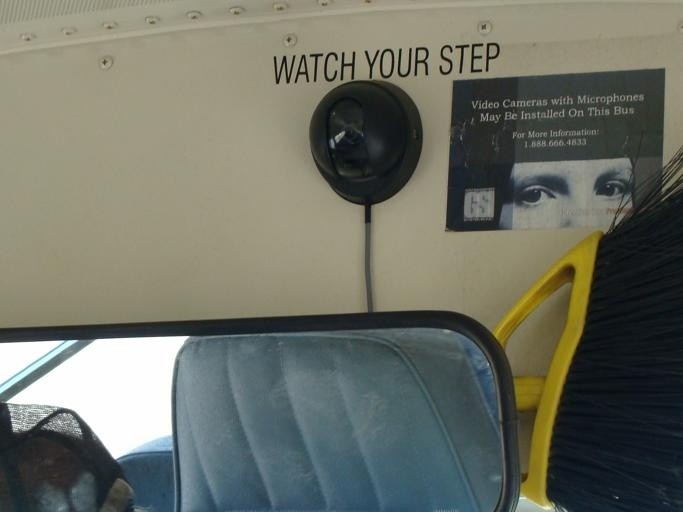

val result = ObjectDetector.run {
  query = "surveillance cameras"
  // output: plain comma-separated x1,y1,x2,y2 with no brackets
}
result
308,79,423,206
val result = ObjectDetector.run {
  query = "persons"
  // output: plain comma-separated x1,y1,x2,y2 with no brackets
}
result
453,157,635,231
